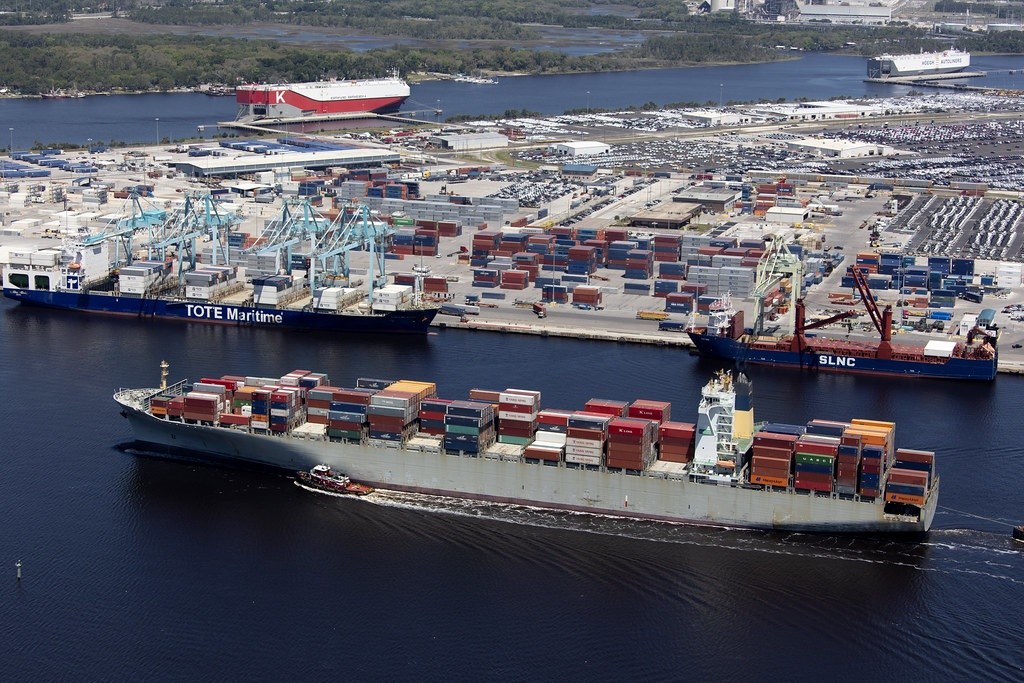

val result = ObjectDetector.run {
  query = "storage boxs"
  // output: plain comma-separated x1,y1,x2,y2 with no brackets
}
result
0,111,998,371
153,369,936,505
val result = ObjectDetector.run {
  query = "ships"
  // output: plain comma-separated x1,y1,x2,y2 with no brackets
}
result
113,367,941,533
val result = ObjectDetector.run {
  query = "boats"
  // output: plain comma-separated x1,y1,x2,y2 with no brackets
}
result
0,188,450,334
236,67,410,123
40,87,85,98
295,463,375,496
867,46,971,77
686,263,998,379
